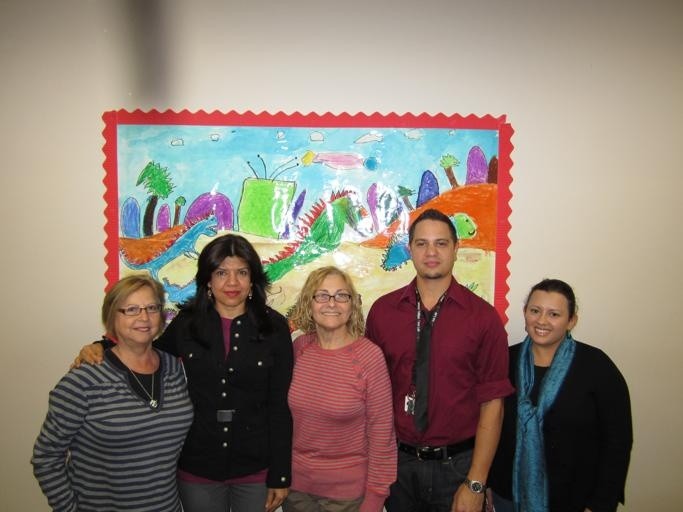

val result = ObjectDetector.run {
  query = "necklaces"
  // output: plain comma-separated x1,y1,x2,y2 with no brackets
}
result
117,347,159,410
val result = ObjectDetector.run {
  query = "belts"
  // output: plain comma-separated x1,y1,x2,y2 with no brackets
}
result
398,435,476,462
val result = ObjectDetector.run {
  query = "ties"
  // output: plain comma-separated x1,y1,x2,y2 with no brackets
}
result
413,309,433,432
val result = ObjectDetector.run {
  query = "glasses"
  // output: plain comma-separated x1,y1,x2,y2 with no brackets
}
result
312,293,352,303
118,303,162,317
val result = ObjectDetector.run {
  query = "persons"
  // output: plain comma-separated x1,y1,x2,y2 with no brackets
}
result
67,235,293,512
364,209,516,512
31,274,195,512
287,266,397,511
484,278,634,512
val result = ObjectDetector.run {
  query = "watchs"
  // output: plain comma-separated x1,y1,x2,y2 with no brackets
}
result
463,478,487,495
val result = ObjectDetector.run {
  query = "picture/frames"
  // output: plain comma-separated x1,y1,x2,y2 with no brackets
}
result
99,108,515,337
